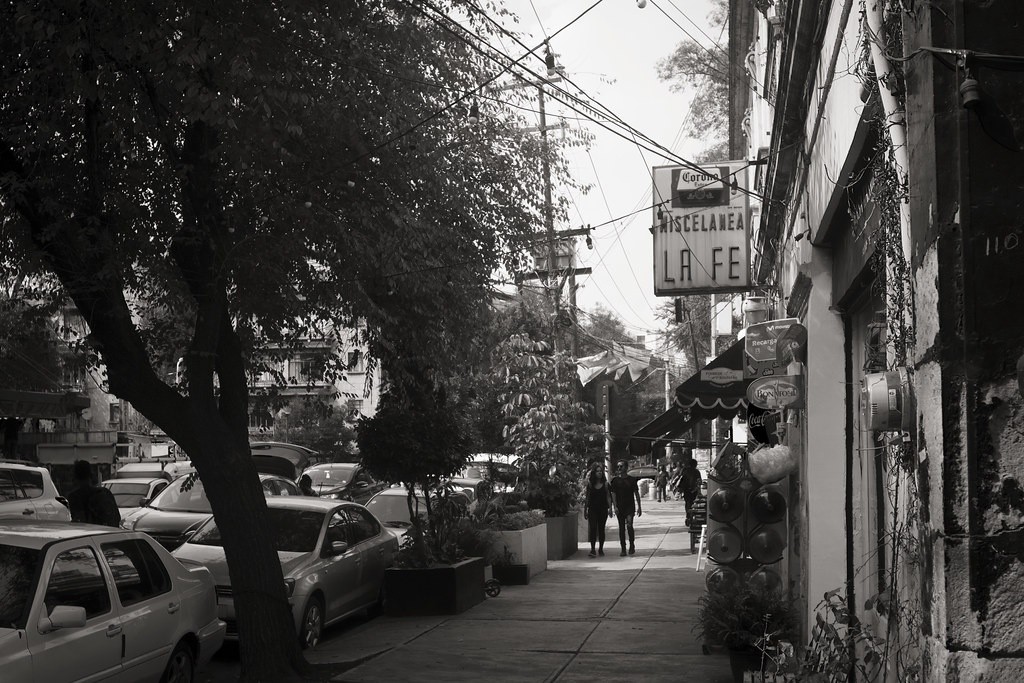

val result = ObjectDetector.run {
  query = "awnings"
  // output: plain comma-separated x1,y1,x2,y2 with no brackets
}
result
673,317,806,419
627,404,704,457
0,389,91,414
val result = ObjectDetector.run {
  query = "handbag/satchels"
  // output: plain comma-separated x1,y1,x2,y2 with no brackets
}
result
672,475,684,492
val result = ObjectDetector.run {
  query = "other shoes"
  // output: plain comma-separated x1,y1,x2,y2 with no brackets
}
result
588,550,597,557
657,500,661,503
628,548,635,555
598,549,605,557
620,551,627,556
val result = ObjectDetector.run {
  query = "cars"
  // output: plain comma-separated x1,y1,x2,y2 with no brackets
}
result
168,495,400,652
100,443,523,551
0,520,225,683
627,468,664,480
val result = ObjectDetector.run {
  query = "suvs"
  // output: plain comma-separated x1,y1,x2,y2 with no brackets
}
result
1,459,72,525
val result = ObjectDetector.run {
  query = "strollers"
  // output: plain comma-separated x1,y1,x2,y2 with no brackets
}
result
666,480,680,500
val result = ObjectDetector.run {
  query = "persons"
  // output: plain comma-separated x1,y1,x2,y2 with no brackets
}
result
654,459,702,527
611,459,642,557
299,474,318,496
64,460,121,528
583,462,612,557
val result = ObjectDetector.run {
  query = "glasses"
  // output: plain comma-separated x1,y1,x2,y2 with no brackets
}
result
616,464,625,468
